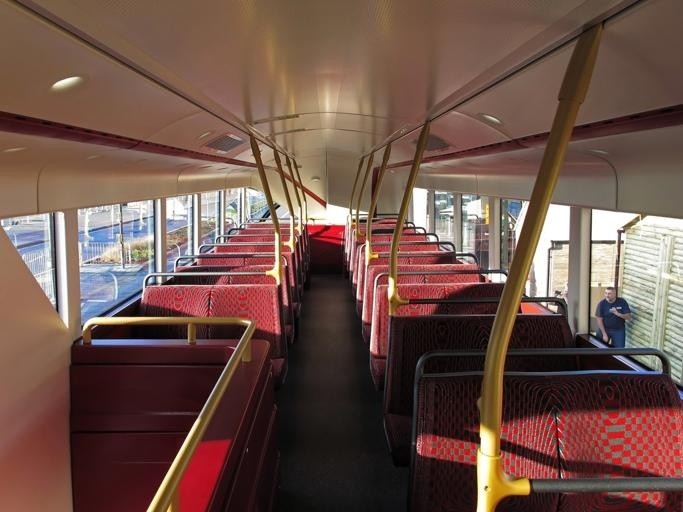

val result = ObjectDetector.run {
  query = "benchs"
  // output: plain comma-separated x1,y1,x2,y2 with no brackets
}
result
344,215,683,512
138,218,310,390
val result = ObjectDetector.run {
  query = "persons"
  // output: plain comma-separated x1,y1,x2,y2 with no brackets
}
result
592,286,632,351
